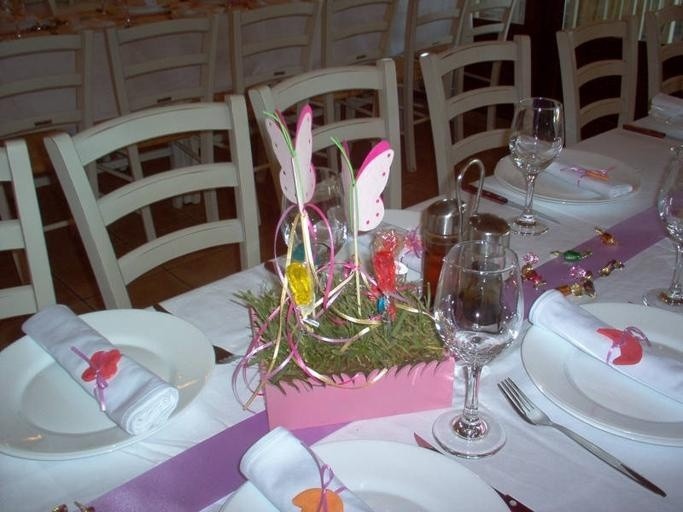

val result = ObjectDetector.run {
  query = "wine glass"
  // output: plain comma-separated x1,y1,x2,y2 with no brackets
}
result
509,96,565,238
643,143,682,312
431,243,523,454
1,0,25,39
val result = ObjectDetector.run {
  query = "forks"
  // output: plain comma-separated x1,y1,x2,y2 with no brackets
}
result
151,301,244,365
495,377,667,497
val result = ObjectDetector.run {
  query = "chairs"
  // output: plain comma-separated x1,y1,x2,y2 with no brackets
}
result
0,1,683,344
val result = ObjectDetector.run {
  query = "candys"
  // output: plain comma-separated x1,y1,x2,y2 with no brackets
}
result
592,223,615,244
520,249,624,296
286,261,316,311
375,233,397,298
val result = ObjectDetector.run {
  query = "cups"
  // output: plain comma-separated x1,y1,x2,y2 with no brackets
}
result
281,168,348,272
100,1,128,30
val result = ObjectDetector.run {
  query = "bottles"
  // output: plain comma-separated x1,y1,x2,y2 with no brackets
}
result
419,199,466,307
465,214,508,326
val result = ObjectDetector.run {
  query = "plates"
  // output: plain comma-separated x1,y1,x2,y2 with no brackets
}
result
492,146,644,206
523,303,683,452
1,308,215,459
361,207,421,283
219,438,512,512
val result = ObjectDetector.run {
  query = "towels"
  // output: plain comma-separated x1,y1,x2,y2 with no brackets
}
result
21,303,180,436
239,426,374,512
528,288,683,405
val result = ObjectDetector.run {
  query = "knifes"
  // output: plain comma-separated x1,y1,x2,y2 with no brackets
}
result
461,183,560,224
623,124,683,142
413,431,535,512
